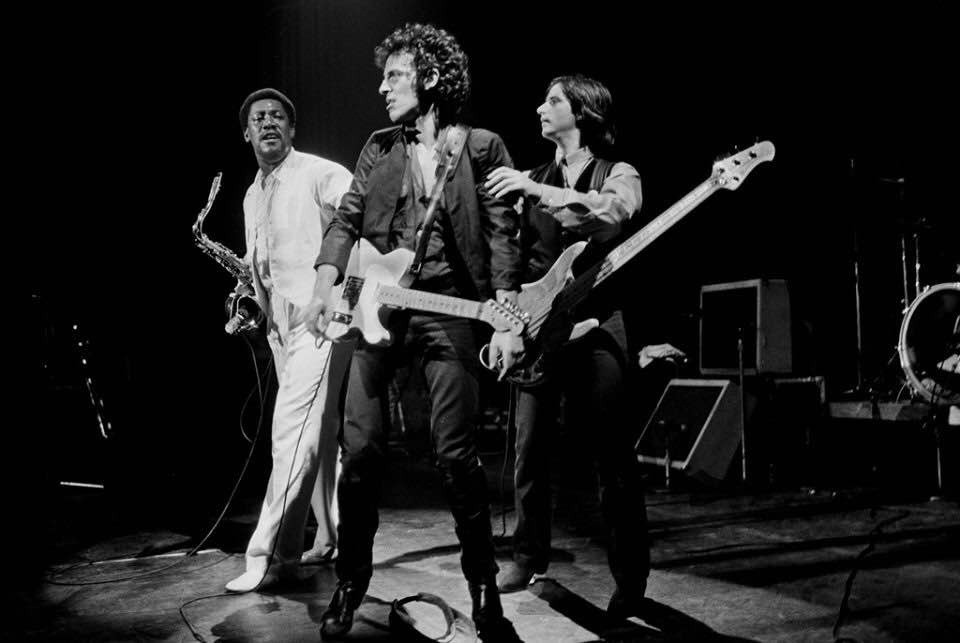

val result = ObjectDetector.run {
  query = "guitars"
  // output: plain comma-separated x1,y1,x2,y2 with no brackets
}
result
314,238,533,345
516,141,776,351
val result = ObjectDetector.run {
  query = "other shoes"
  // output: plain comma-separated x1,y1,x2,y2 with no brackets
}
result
320,575,371,642
607,578,648,618
300,544,338,564
498,552,549,592
226,571,265,594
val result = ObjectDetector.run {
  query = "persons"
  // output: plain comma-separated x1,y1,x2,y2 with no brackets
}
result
483,76,653,617
304,25,526,643
224,88,382,597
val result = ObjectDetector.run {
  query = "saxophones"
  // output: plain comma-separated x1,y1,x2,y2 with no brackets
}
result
194,172,265,333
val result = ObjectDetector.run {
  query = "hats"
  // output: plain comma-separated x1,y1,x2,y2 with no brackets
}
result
387,591,478,643
238,88,297,130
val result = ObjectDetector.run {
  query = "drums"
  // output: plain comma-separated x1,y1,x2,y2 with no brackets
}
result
898,282,960,404
